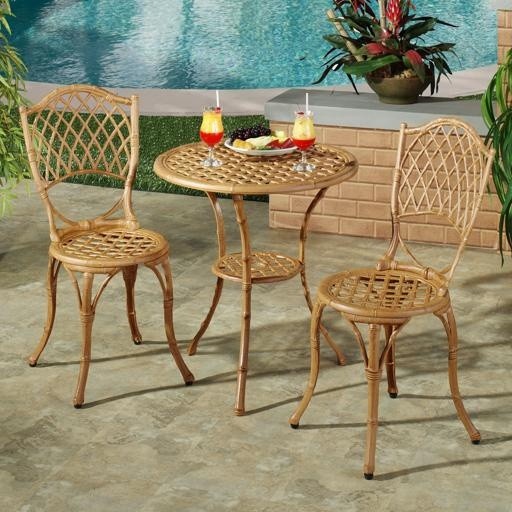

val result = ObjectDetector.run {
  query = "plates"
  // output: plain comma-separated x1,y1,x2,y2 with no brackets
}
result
224,134,299,158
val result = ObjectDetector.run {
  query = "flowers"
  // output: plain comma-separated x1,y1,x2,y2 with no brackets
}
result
310,0,462,104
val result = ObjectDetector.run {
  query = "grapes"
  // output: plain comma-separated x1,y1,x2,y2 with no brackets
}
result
230,123,271,142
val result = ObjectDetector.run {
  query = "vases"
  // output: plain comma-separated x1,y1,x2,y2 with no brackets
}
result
362,69,434,104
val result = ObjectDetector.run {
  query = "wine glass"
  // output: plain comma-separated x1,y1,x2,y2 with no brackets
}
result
292,110,317,172
199,105,225,168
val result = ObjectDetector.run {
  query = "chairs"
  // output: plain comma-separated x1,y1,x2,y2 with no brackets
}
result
18,83,197,410
286,116,499,482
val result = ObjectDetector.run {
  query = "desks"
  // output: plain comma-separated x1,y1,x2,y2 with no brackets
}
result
151,135,363,418
